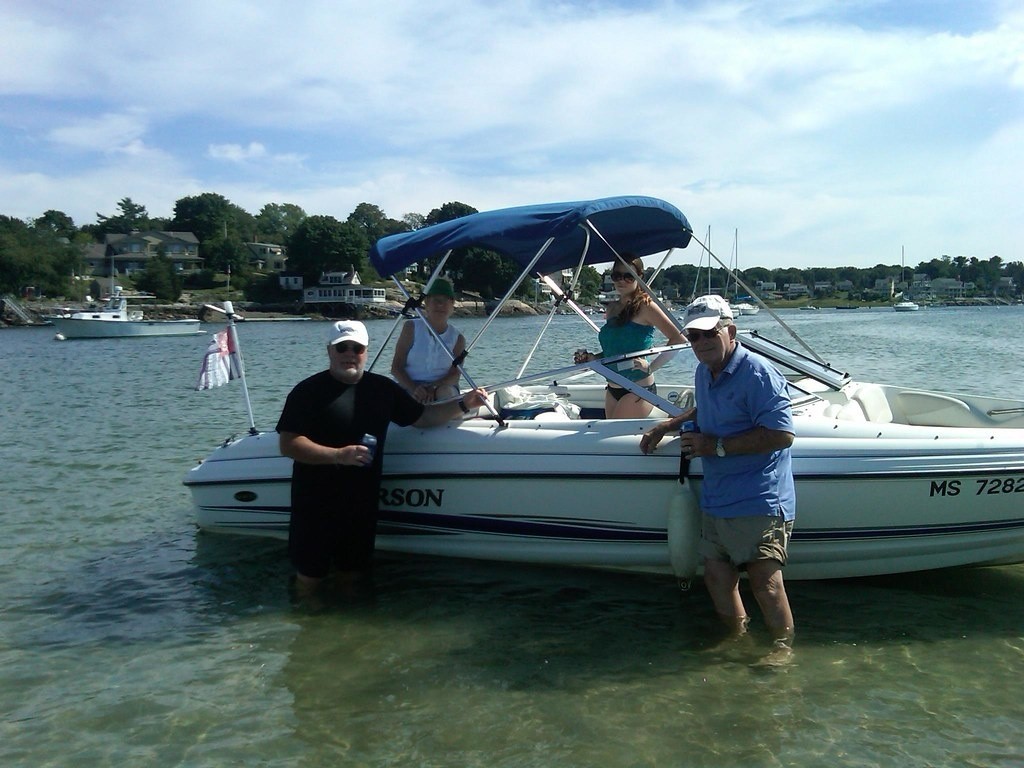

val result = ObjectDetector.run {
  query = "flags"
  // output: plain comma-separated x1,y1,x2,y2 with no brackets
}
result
194,326,241,392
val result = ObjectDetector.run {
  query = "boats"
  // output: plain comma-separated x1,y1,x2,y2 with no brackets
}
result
894,302,919,312
180,196,1023,572
53,309,203,340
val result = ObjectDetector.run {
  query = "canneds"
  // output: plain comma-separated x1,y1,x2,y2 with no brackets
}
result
362,434,377,464
577,349,588,363
680,420,699,434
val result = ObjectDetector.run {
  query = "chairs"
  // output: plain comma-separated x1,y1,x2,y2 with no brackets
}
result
832,383,896,425
533,411,570,423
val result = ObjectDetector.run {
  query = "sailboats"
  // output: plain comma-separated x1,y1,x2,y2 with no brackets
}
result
687,225,759,318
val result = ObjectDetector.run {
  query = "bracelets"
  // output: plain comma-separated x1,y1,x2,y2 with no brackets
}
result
456,397,470,414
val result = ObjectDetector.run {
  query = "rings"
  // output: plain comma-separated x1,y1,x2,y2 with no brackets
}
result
688,446,691,452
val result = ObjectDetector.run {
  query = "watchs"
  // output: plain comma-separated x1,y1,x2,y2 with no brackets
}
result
716,437,726,458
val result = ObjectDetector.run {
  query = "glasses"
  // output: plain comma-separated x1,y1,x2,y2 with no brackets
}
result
332,342,365,355
686,324,729,342
610,271,636,283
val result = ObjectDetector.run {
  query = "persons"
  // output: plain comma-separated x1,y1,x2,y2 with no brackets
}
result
573,255,687,420
639,295,796,669
392,276,466,404
276,321,490,602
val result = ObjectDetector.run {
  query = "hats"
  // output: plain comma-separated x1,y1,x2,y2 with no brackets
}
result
326,319,369,347
679,295,733,335
428,278,457,298
